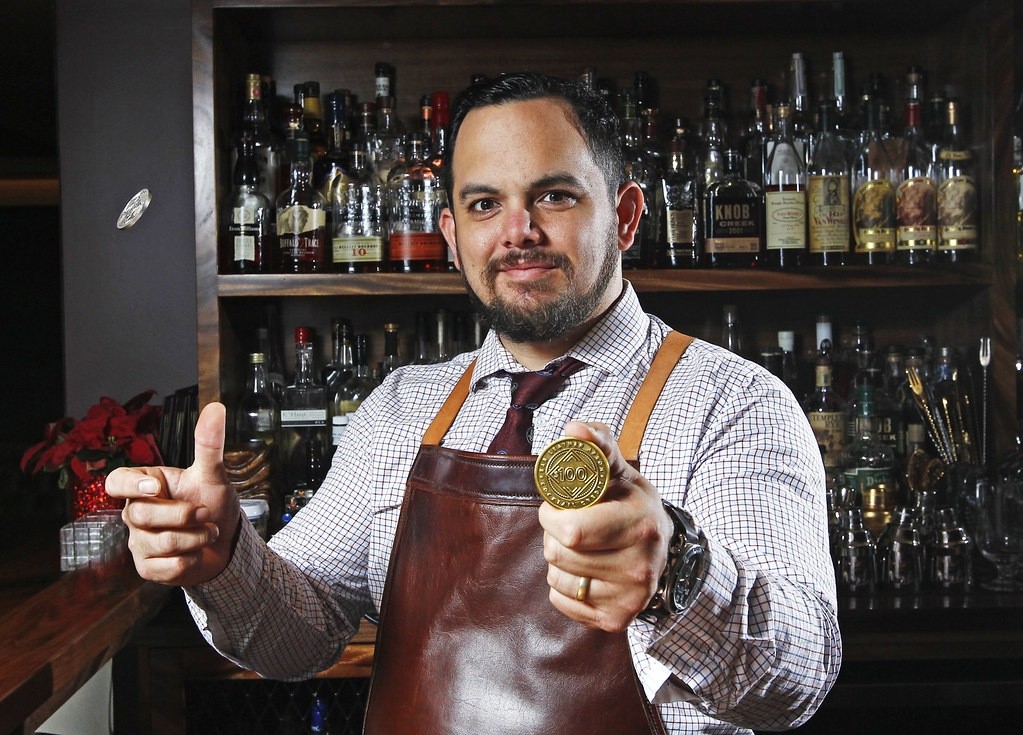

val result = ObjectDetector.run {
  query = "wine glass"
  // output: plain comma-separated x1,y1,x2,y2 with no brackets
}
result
975,478,1023,605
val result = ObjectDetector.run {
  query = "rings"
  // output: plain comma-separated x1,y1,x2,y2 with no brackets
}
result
576,576,591,603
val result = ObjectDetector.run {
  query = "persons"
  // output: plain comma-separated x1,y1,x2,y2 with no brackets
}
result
105,69,843,735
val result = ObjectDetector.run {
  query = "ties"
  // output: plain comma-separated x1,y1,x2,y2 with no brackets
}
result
486,356,588,456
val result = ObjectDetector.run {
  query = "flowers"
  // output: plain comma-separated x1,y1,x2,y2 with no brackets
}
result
21,388,166,489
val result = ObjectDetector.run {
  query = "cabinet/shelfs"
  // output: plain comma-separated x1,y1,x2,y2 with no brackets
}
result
188,0,1023,735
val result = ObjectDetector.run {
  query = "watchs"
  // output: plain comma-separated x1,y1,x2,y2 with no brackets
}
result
634,499,707,628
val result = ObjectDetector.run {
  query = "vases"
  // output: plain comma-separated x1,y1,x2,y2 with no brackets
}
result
72,470,126,520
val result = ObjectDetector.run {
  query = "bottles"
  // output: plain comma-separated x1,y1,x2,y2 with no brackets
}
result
221,51,1022,600
